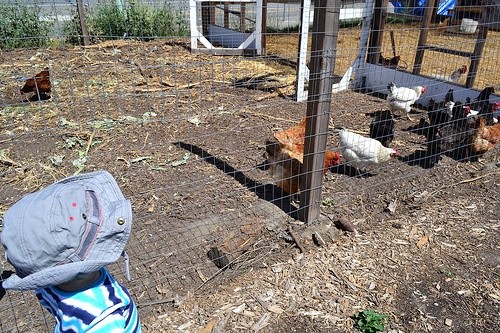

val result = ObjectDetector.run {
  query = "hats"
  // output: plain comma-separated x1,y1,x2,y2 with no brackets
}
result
0,170,132,292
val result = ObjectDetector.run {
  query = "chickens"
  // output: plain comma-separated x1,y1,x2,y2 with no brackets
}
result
416,86,500,168
379,52,400,70
387,80,426,121
20,67,52,95
268,115,306,145
262,140,340,203
433,17,450,36
451,65,468,83
336,126,402,180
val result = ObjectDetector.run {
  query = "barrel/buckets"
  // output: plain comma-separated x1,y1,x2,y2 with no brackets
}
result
464,21,477,32
460,18,472,30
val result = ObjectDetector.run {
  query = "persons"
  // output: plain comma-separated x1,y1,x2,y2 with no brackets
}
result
0,168,145,333
